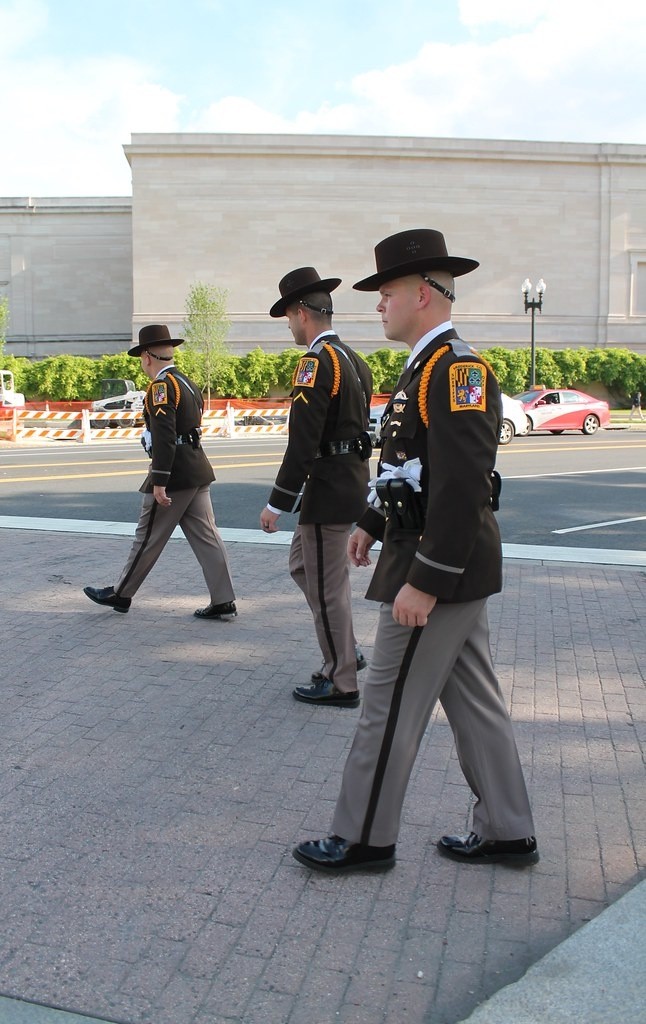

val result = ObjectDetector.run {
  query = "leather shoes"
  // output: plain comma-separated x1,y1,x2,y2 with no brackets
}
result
291,678,361,708
194,601,237,619
436,830,540,867
84,586,131,613
310,647,367,682
291,834,396,872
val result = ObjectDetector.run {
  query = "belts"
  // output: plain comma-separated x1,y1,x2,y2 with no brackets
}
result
310,434,361,460
176,435,195,446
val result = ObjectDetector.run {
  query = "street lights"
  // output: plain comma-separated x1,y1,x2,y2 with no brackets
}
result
520,278,548,386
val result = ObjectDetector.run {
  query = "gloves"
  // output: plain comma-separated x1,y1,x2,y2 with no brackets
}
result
367,456,422,508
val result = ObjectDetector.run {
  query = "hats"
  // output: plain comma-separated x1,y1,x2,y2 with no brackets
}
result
270,266,342,317
353,230,480,291
129,324,185,357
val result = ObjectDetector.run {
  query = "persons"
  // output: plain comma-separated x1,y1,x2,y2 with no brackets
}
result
629,392,644,420
289,230,539,873
260,266,374,707
84,326,239,619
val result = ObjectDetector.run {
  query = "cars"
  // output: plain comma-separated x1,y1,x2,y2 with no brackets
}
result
366,393,528,446
508,383,612,436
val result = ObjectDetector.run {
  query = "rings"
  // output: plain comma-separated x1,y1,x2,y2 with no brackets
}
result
265,523,269,528
